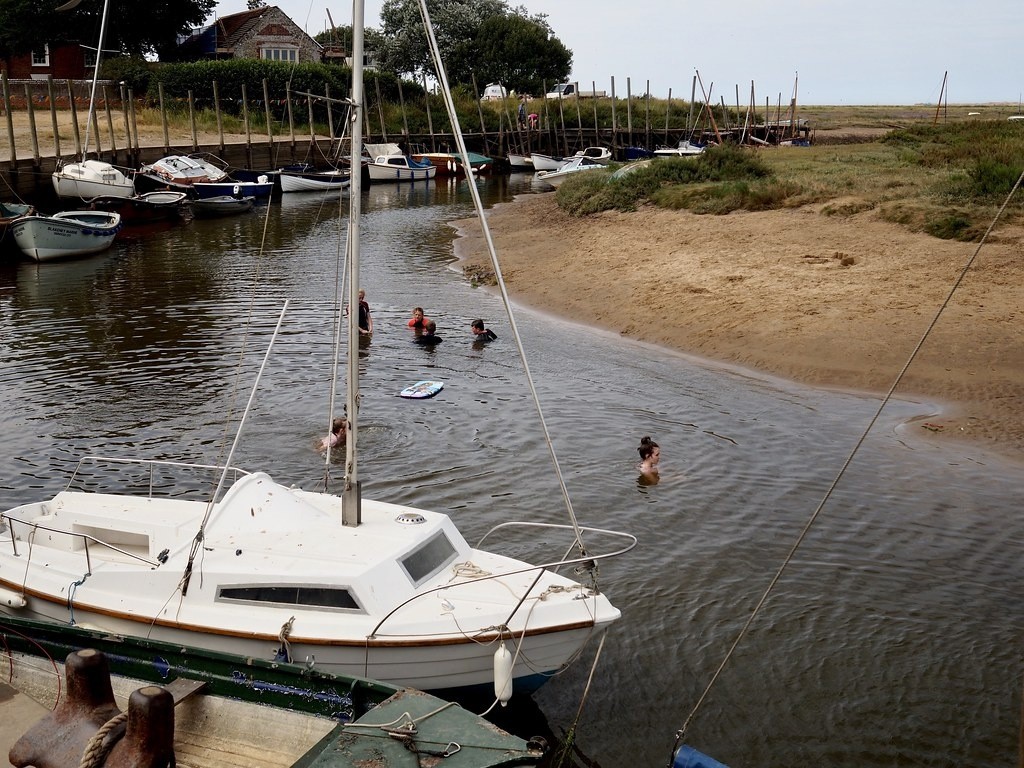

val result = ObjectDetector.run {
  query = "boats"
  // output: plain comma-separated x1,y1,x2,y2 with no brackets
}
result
0,203,121,263
369,181,437,198
282,191,351,216
0,618,550,768
15,254,118,311
0,0,635,710
189,196,256,218
232,144,492,192
193,175,274,198
140,152,229,195
508,139,809,190
78,192,187,222
52,1,137,202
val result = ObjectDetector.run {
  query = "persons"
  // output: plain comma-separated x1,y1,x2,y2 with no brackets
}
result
408,307,430,327
637,435,661,485
346,289,374,335
516,101,527,129
471,320,497,341
528,114,539,130
321,416,347,451
413,321,443,344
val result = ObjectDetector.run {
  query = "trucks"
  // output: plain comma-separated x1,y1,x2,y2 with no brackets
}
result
544,84,606,100
